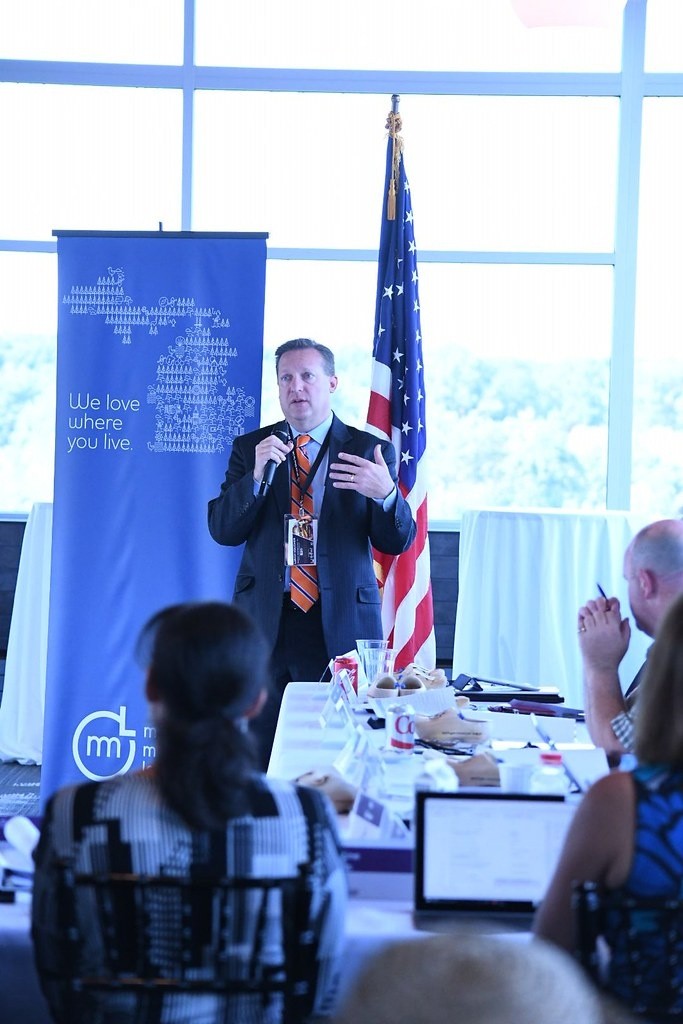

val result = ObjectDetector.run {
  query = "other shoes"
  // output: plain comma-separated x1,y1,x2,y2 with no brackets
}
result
446,750,500,788
292,771,357,814
399,663,445,677
398,669,447,689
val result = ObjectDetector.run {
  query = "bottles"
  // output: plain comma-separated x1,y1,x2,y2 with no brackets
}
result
530,754,571,798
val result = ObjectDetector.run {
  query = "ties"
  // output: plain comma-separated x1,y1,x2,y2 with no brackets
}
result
290,435,319,613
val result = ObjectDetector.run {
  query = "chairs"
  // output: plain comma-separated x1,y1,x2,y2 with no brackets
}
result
70,878,317,1024
573,880,683,1024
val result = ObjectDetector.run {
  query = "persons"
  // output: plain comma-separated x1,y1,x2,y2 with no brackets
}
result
208,341,416,775
340,931,607,1024
30,603,350,1024
534,594,683,1023
578,520,683,770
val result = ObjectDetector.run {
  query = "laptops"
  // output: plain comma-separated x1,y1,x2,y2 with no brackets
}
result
411,787,580,938
529,711,591,794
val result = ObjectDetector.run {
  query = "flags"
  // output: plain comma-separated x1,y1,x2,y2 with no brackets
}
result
365,113,437,675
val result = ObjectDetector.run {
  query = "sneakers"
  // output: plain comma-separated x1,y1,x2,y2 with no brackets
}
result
367,674,398,699
414,707,490,744
399,674,426,696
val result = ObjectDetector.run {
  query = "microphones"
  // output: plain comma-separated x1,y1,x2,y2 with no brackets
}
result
259,422,290,497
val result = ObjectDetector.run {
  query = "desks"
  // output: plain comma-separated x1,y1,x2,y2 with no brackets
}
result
452,507,655,711
266,682,597,835
0,902,534,1024
0,502,54,765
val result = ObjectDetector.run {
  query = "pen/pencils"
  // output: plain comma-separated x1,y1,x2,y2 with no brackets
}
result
594,583,607,601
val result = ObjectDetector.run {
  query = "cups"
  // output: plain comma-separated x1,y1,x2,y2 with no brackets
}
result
355,639,389,672
364,648,397,687
